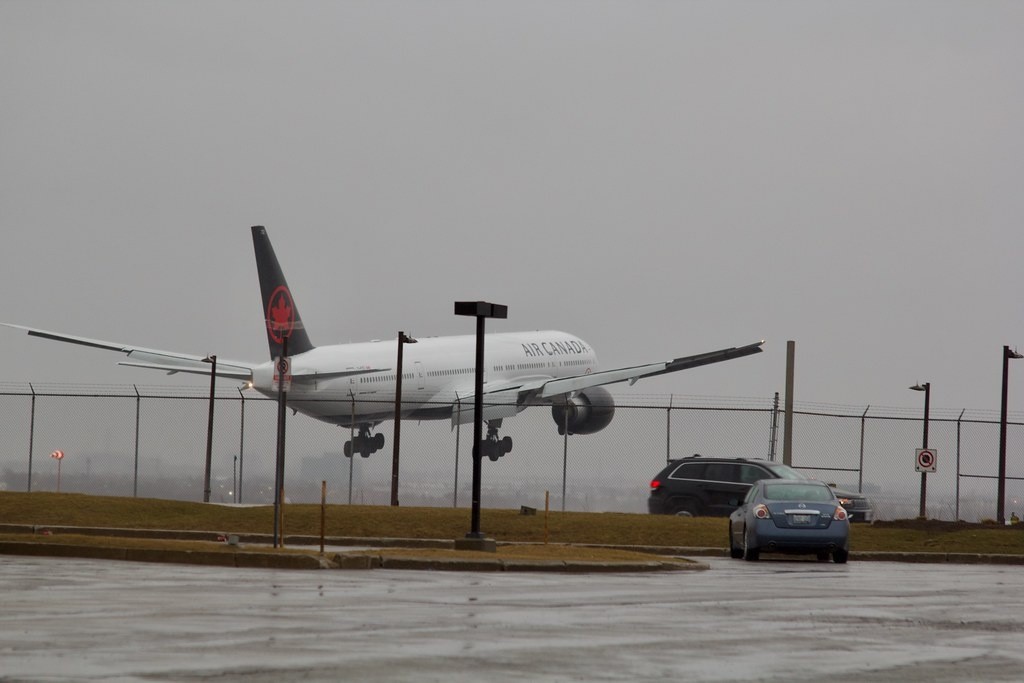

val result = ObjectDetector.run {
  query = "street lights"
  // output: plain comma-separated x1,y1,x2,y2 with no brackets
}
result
996,345,1024,524
390,330,418,507
453,299,509,552
909,382,932,523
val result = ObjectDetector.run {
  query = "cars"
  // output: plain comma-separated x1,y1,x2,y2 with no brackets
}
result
729,478,851,564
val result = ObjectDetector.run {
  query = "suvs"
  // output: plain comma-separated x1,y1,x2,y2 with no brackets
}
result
649,454,872,525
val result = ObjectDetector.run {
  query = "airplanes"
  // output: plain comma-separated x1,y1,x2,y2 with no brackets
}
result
0,224,766,459
